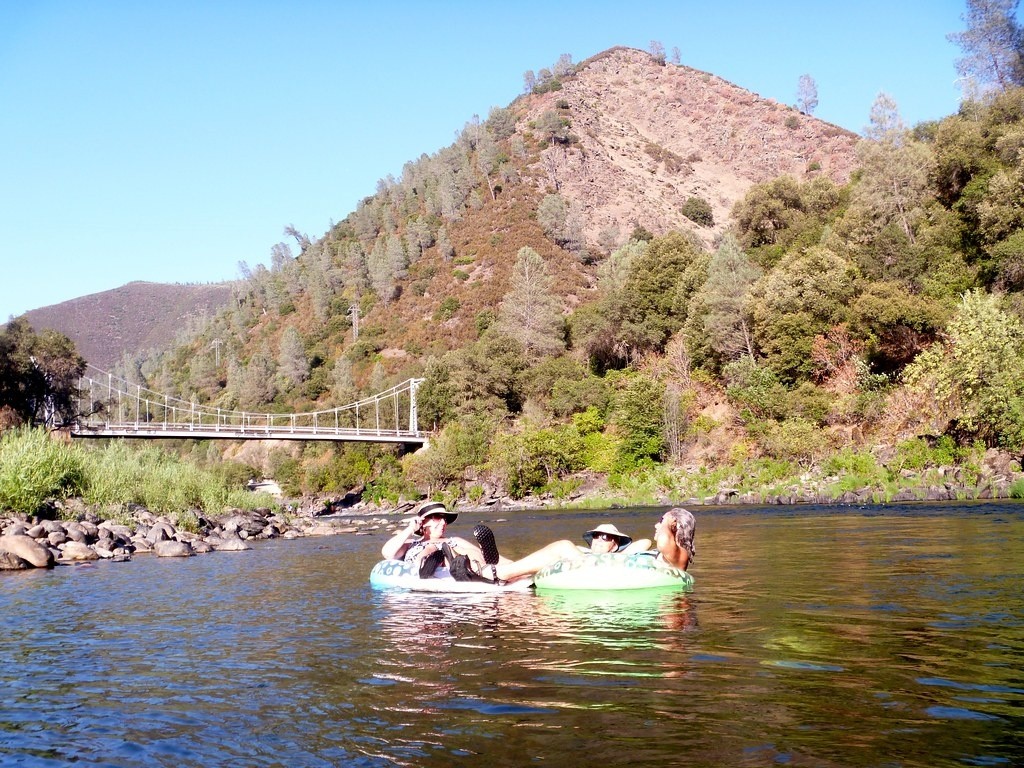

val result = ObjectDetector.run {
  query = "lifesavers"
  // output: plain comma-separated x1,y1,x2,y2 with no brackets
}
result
534,552,696,590
369,559,534,594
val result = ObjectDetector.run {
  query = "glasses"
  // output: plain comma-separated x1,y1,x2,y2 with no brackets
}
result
593,532,613,541
658,517,663,523
428,514,451,524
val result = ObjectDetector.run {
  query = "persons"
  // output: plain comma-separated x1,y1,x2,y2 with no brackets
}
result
381,501,499,580
441,508,697,587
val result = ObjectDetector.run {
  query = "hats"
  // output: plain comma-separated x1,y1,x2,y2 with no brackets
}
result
414,501,460,536
583,524,633,550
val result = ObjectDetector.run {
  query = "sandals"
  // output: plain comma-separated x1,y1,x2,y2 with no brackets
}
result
464,558,508,585
449,554,469,580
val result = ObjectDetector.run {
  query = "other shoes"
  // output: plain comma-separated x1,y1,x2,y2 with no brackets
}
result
474,523,500,564
442,543,458,564
420,550,444,578
